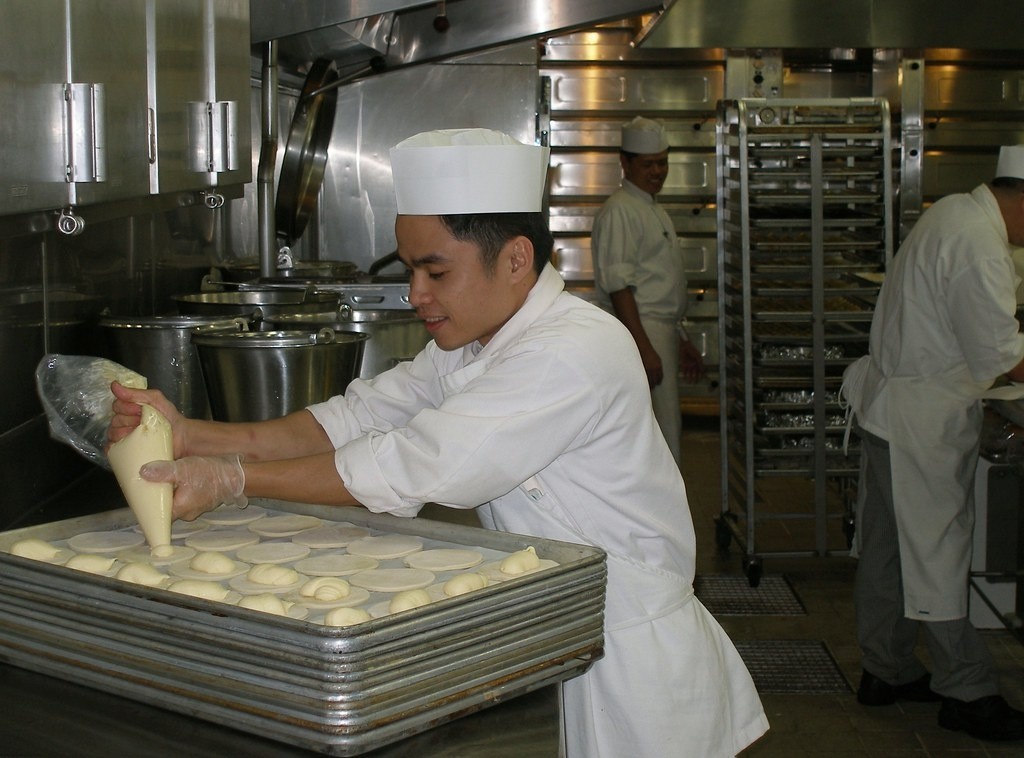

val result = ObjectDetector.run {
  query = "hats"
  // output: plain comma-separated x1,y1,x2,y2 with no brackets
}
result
622,116,669,155
390,128,551,215
995,144,1024,179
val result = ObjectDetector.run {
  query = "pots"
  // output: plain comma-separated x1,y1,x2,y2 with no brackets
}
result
260,303,436,381
189,327,372,423
84,316,246,422
214,246,357,283
0,281,113,433
170,275,342,333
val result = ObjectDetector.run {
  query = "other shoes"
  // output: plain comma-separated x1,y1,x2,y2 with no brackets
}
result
857,669,945,708
938,696,1024,743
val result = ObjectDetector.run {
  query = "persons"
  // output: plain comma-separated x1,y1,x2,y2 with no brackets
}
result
103,127,771,758
590,115,708,465
838,144,1024,742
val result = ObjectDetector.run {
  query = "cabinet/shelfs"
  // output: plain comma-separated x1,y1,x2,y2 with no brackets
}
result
714,95,894,580
0,0,252,228
